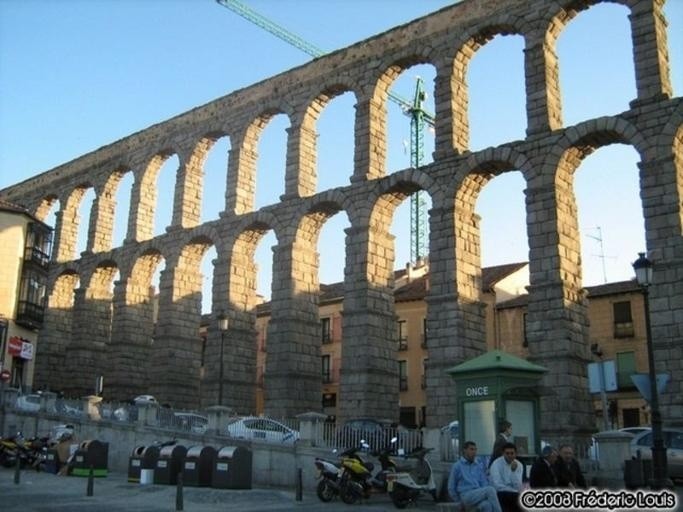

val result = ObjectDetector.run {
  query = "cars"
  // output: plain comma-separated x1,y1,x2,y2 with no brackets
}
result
443,418,552,456
630,427,683,477
173,411,209,432
76,404,110,420
133,391,154,403
15,394,78,418
587,425,651,461
338,418,420,448
205,416,300,446
37,390,57,400
204,404,238,422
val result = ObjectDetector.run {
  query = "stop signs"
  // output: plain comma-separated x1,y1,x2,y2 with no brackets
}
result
0,370,12,382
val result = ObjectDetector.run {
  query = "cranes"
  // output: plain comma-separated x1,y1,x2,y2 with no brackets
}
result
217,2,434,268
583,222,618,283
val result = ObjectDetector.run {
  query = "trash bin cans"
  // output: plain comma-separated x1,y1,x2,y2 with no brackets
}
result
46,434,110,478
127,445,253,490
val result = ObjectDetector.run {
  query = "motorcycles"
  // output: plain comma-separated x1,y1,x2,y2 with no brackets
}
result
341,437,399,501
315,440,369,500
381,444,439,508
0,431,58,473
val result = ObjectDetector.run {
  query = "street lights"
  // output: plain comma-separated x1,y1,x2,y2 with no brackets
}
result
216,309,228,407
631,251,670,491
591,343,611,430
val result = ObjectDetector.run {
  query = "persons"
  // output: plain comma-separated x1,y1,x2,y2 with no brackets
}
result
489,442,524,512
489,422,513,467
448,442,503,512
530,446,575,488
556,444,587,489
